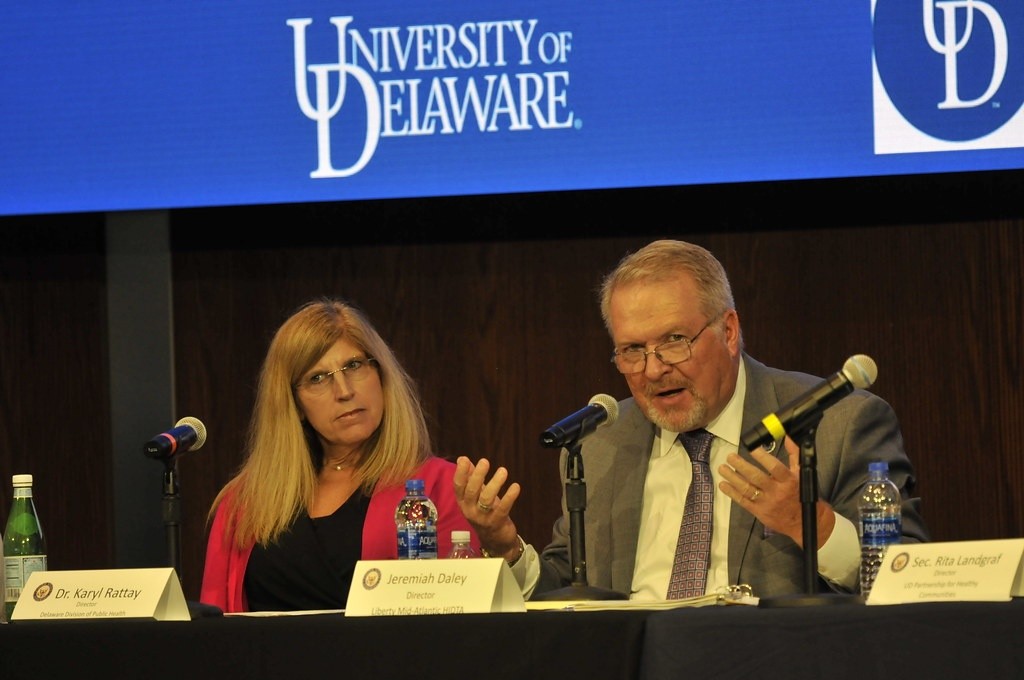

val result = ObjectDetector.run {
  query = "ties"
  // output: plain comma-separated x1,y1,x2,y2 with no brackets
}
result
666,427,716,600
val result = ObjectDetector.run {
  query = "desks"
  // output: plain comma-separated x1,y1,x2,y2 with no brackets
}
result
0,596,1024,680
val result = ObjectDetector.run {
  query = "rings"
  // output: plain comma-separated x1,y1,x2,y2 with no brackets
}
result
477,500,492,513
750,489,760,501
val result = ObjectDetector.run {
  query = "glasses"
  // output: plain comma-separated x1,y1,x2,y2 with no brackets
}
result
295,358,375,396
609,308,727,374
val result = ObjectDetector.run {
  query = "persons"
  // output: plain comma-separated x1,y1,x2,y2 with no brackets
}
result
199,300,483,614
453,239,932,601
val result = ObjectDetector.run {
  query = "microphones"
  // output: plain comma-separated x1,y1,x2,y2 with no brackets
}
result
143,417,207,460
741,354,878,453
540,393,619,448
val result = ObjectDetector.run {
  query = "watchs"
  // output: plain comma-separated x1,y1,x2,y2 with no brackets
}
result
479,537,523,567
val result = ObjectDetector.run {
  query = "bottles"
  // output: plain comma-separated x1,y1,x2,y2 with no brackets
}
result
2,474,47,621
394,480,437,560
858,462,901,598
446,530,478,558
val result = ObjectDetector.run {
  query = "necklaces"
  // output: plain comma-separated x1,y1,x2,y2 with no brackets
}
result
324,460,356,471
